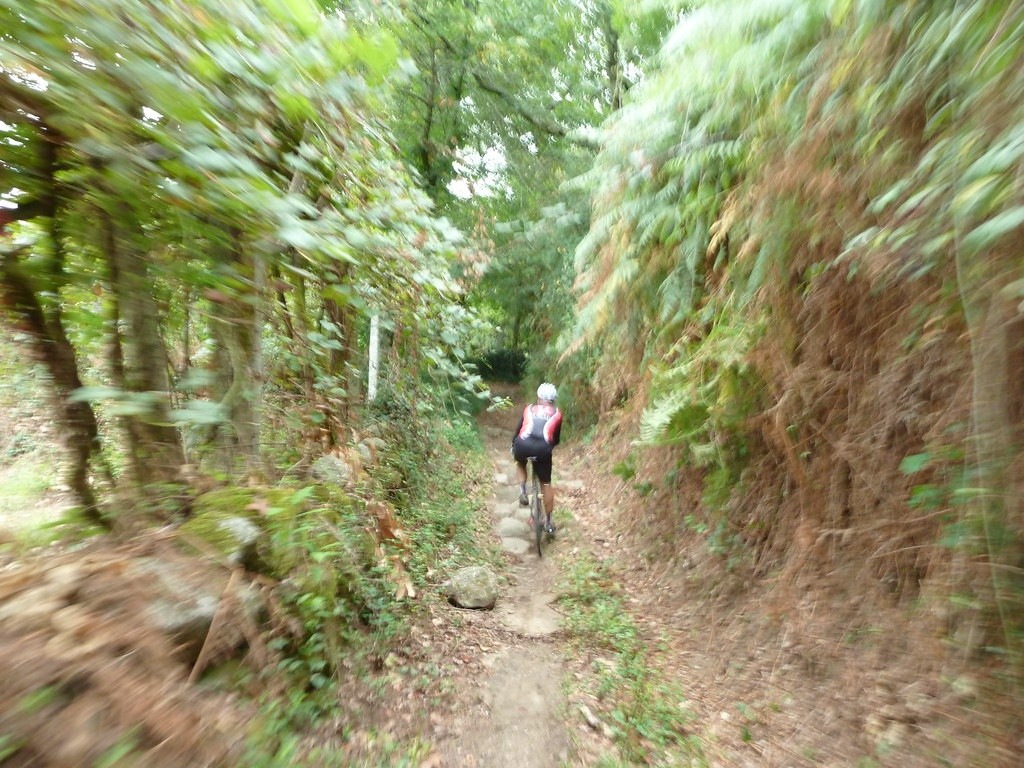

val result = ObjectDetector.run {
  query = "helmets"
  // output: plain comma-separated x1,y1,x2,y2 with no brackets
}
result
537,383,556,400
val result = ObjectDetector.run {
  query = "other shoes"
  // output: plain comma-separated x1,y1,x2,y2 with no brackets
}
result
518,492,529,508
543,521,556,543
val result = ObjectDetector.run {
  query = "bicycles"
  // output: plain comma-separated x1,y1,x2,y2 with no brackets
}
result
509,443,557,558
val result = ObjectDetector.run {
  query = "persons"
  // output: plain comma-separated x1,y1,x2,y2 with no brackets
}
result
509,381,565,557
